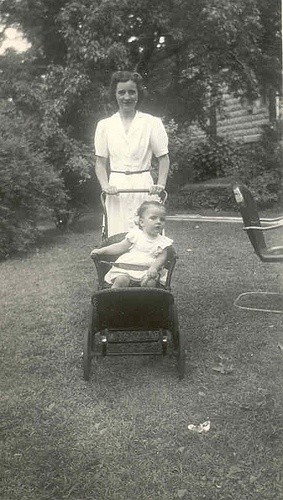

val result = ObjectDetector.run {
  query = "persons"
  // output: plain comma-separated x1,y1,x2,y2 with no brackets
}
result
91,200,173,289
94,70,170,233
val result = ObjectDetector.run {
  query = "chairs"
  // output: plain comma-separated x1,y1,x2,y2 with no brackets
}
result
231,186,283,313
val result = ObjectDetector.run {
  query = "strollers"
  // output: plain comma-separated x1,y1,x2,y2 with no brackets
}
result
82,186,186,380
